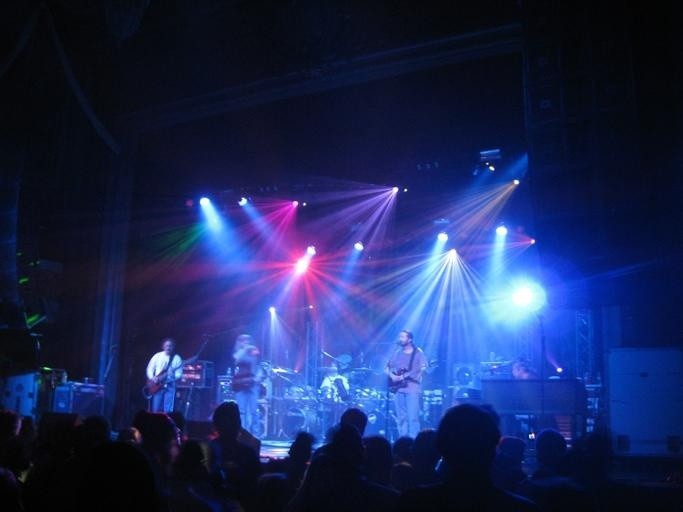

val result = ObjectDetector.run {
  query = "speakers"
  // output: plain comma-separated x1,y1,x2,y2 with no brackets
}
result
54,382,104,418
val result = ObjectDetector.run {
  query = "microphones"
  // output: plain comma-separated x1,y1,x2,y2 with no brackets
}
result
321,350,331,358
392,341,402,344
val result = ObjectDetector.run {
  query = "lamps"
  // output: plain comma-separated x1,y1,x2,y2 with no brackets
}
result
465,139,503,176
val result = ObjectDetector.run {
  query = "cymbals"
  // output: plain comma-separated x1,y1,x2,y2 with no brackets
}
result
270,368,288,373
315,367,335,373
352,367,376,373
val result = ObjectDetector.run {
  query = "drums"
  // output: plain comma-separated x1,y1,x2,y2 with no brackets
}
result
287,387,304,407
279,407,319,441
320,386,335,403
364,409,400,443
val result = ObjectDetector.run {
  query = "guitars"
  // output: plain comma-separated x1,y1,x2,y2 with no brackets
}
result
145,355,198,394
232,373,273,392
387,360,437,393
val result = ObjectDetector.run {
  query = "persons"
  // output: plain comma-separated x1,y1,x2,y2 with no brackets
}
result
230,333,264,438
1,402,681,512
382,329,430,442
510,360,560,438
317,362,350,402
144,339,183,413
